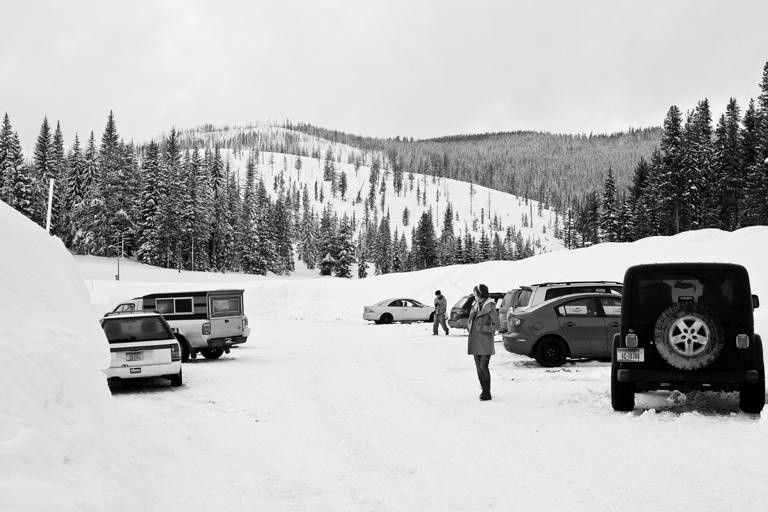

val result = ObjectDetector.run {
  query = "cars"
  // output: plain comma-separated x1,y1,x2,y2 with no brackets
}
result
498,289,522,333
503,293,622,367
363,297,449,324
98,309,183,393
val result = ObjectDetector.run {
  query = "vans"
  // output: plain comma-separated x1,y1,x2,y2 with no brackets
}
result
112,289,251,363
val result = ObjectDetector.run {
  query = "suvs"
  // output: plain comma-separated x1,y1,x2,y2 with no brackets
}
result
507,281,623,325
448,292,506,334
611,262,765,413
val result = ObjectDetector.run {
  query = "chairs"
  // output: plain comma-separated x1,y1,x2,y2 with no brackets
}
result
642,281,734,310
103,319,168,341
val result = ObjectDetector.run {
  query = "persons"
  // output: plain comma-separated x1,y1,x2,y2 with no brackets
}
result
466,283,501,400
432,290,449,336
